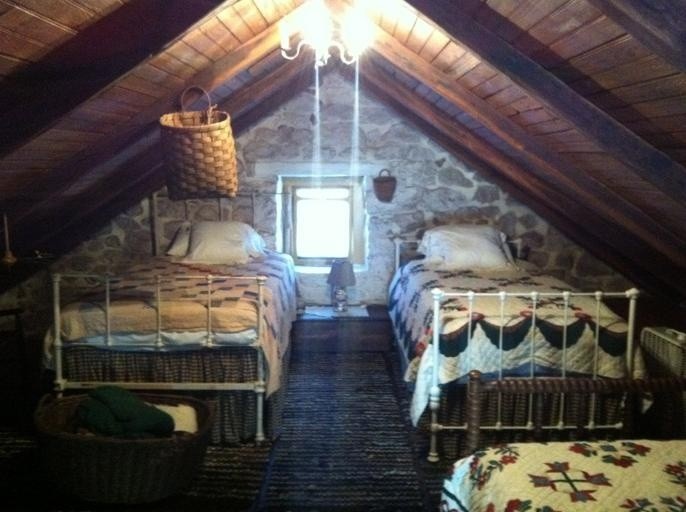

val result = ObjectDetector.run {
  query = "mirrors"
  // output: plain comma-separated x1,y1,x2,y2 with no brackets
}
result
416,224,519,272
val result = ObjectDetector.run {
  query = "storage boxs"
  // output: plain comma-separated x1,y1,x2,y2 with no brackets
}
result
293,306,390,354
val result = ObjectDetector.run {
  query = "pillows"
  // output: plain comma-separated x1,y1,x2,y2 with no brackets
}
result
166,221,191,257
181,220,264,265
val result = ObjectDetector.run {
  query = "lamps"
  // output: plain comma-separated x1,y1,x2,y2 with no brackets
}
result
278,0,376,69
327,262,356,312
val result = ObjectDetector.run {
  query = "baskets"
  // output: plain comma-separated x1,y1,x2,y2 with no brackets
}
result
33,393,216,504
158,111,238,200
373,169,395,201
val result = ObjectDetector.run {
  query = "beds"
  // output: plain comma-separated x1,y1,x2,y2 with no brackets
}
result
40,193,302,446
387,238,655,463
439,439,686,512
